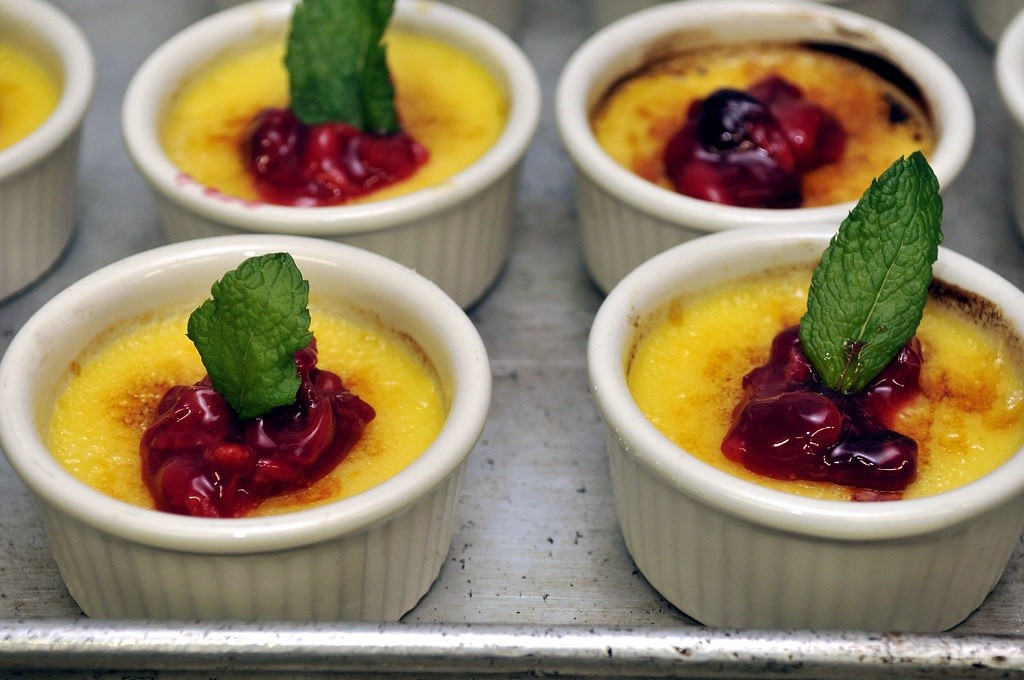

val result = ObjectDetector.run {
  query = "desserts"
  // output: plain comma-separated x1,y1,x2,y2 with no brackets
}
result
552,0,976,297
587,150,1024,632
0,234,494,624
0,0,96,299
119,0,543,313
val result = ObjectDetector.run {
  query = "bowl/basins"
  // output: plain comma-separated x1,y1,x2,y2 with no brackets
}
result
0,0,99,298
120,0,543,314
588,227,1024,631
0,234,493,620
995,11,1024,230
556,0,978,296
